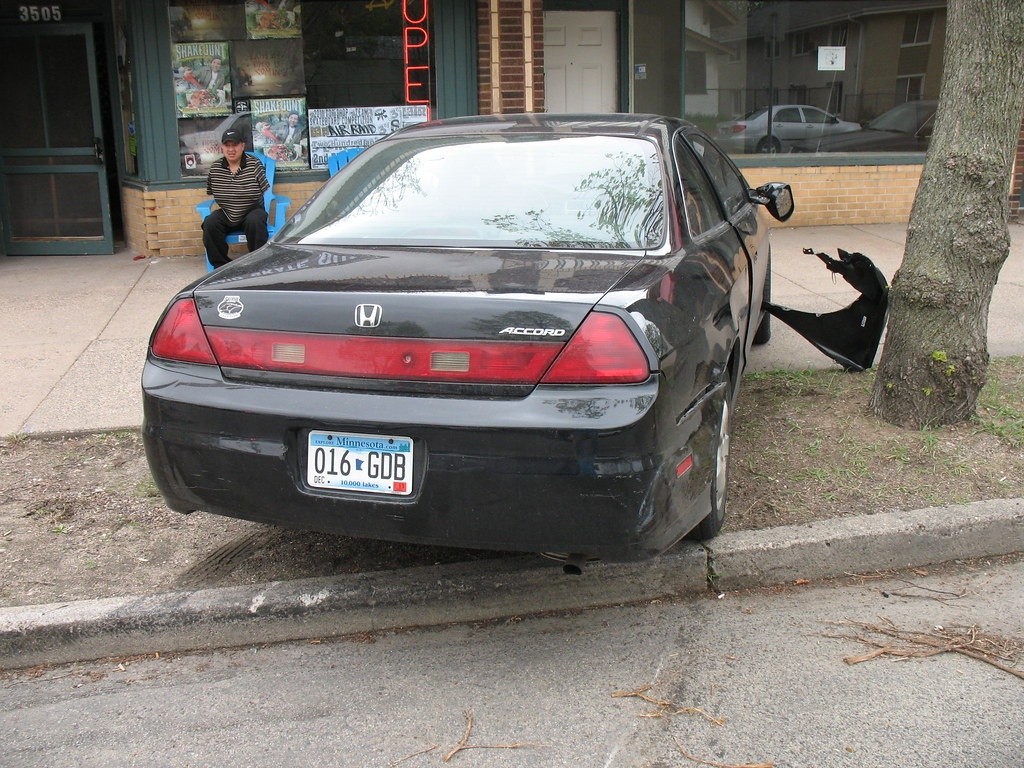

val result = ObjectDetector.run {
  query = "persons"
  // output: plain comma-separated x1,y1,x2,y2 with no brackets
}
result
262,112,302,150
184,56,225,90
199,128,270,270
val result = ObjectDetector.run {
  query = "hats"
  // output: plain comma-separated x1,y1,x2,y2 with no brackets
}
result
221,128,241,144
288,110,300,118
210,55,223,63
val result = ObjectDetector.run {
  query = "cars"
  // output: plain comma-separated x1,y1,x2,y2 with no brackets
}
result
175,111,253,174
789,98,939,152
712,105,863,155
141,112,798,563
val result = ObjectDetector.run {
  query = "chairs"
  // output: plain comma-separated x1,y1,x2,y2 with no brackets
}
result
196,151,291,274
327,148,367,178
